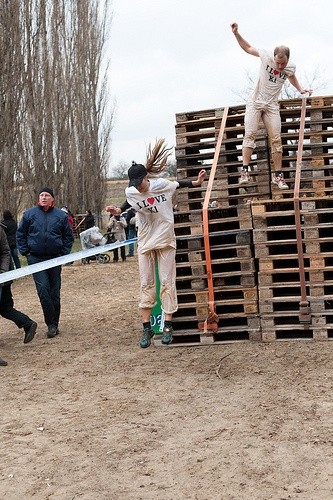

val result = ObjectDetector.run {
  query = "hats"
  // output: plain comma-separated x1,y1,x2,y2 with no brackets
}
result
128,163,147,187
39,187,54,198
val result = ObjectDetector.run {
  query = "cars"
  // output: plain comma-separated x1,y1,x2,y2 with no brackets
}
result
121,212,128,219
151,170,171,178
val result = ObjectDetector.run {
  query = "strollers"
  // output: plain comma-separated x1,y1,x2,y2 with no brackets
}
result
79,227,116,264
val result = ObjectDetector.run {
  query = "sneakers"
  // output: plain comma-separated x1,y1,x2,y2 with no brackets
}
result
161,325,174,345
23,320,37,343
47,324,59,338
140,328,155,348
272,173,289,189
238,169,251,184
0,359,7,366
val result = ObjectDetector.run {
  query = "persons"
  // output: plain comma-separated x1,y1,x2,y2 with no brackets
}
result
0,137,206,366
230,22,311,191
